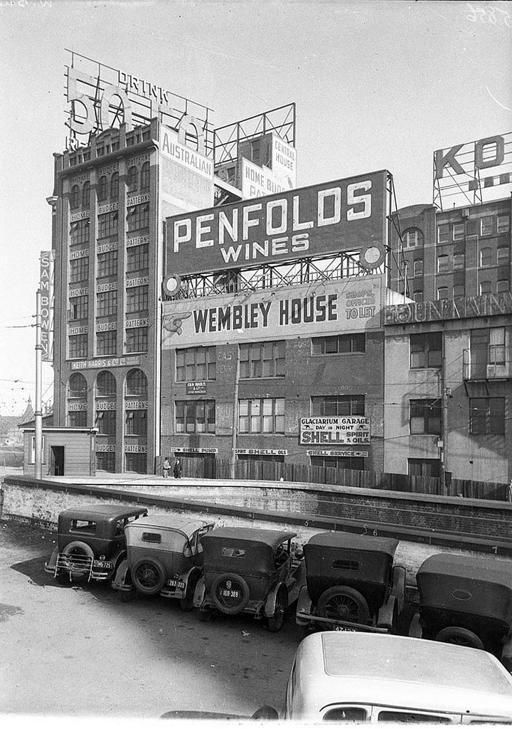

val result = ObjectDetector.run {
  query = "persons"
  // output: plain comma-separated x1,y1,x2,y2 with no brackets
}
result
162,456,182,478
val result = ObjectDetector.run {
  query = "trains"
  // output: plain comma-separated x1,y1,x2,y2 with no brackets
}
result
45,503,511,665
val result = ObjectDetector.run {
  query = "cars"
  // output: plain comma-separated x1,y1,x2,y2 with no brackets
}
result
283,631,512,724
